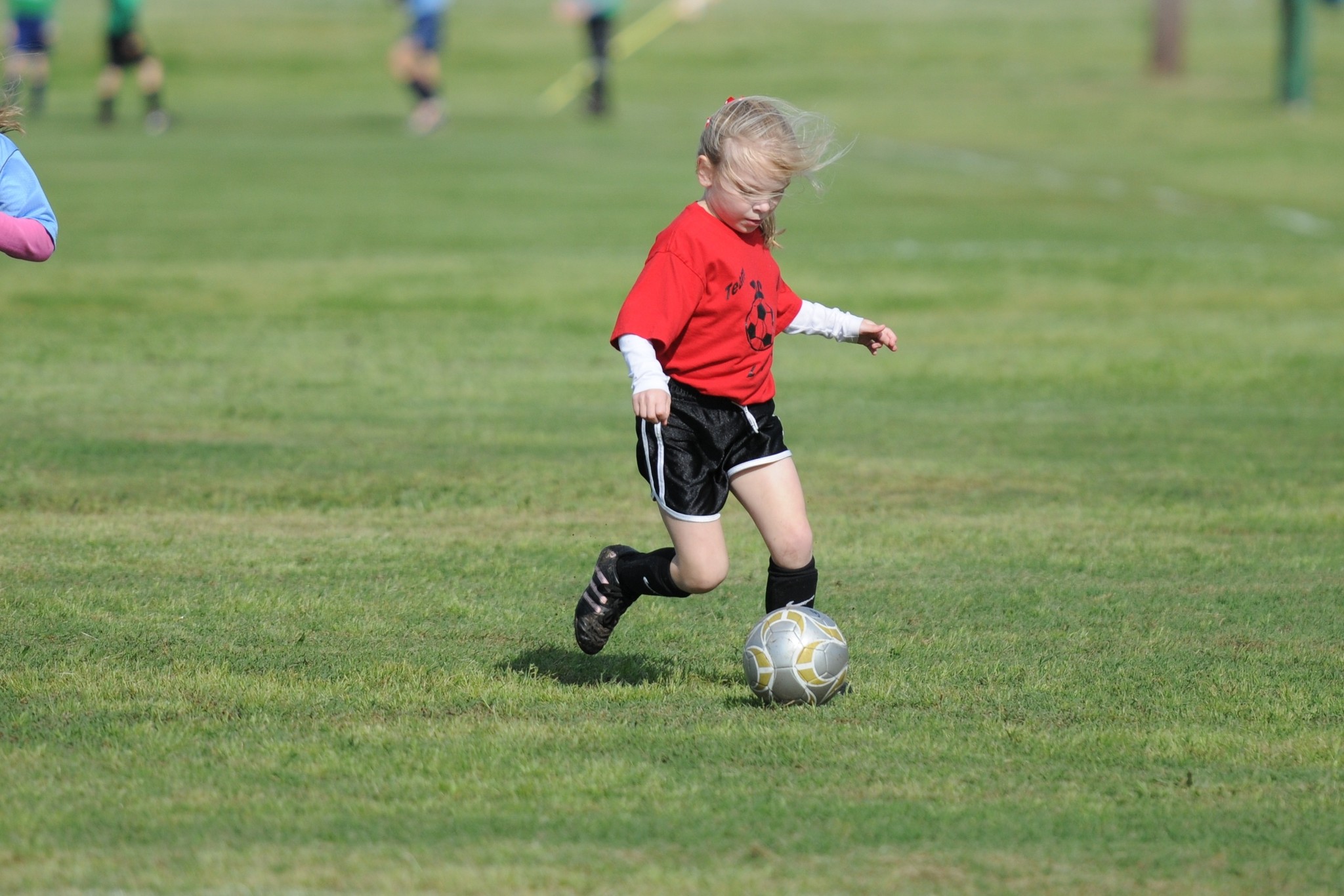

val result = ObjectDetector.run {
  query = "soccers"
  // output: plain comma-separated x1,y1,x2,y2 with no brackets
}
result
744,605,851,709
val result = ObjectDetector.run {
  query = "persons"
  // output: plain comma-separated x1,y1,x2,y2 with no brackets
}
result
98,0,162,125
391,0,448,130
573,96,898,658
0,96,58,262
4,0,52,106
559,0,620,112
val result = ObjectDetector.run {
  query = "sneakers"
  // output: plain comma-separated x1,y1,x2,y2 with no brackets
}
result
573,545,645,657
